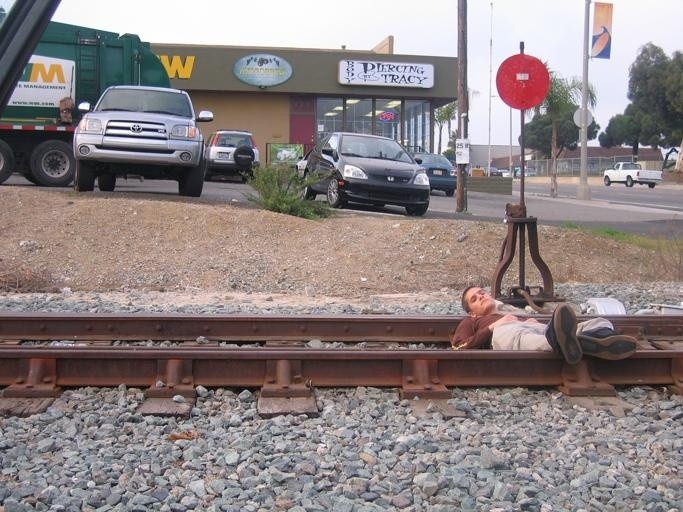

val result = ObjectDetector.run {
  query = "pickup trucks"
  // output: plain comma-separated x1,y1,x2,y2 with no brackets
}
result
603,162,663,188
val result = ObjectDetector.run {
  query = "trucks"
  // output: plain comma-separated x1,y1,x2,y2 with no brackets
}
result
0,13,172,187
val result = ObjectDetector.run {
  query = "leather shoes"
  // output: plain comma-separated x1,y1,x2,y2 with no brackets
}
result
575,327,640,361
543,301,583,365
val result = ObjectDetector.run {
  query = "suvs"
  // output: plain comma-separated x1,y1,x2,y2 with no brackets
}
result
204,130,260,182
72,86,214,197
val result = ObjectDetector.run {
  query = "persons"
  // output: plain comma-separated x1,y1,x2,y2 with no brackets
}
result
451,286,640,365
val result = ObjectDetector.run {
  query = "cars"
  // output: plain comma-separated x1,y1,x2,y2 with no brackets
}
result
468,167,511,177
294,131,457,216
516,168,536,178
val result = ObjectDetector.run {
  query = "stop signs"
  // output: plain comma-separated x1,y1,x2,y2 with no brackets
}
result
496,54,550,111
